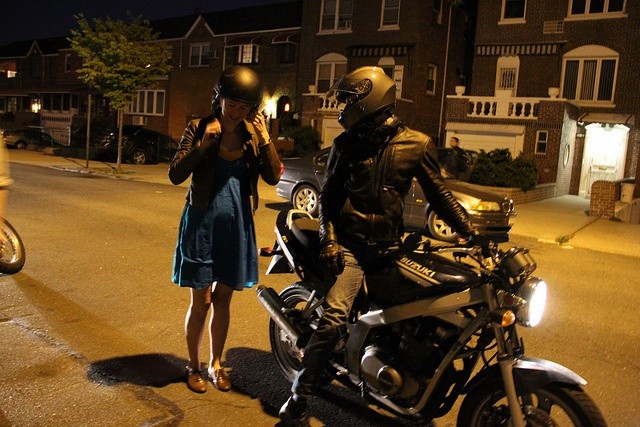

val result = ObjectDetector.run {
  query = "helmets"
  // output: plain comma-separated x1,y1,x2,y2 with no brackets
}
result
210,65,263,119
325,65,397,132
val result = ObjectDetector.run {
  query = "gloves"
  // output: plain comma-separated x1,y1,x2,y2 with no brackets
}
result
318,241,345,275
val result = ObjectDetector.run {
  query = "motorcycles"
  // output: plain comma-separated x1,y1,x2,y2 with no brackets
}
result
0,217,25,275
255,209,608,427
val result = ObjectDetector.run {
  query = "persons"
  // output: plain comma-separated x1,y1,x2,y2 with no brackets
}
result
445,133,467,178
168,66,285,393
278,66,474,421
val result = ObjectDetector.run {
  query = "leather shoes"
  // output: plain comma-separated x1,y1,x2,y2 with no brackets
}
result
278,393,313,427
208,366,232,391
185,362,207,393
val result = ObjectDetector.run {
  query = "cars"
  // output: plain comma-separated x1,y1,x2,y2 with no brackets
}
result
0,128,64,150
276,145,517,242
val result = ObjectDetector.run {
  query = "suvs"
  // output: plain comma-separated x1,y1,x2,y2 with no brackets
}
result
90,125,181,164
